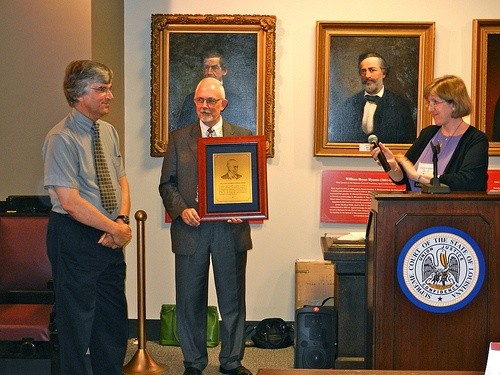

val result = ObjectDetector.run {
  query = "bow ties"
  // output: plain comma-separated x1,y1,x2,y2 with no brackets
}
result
365,95,380,104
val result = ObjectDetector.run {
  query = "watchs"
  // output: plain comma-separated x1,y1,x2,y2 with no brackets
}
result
117,215,129,224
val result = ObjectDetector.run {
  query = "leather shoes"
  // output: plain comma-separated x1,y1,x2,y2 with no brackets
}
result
219,365,253,375
183,367,202,375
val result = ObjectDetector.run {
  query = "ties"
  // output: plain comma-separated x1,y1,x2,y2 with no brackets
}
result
207,128,215,137
91,122,117,214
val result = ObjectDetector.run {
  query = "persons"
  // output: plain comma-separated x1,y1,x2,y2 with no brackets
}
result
369,74,489,194
41,60,131,375
158,78,254,375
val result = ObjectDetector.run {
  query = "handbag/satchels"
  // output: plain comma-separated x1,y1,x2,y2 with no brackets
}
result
158,304,219,348
251,318,293,349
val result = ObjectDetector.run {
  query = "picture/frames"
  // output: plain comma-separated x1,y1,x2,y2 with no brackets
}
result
471,18,500,156
150,14,277,160
313,20,435,158
196,134,270,222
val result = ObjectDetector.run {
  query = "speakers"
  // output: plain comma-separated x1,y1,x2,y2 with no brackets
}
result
294,304,338,369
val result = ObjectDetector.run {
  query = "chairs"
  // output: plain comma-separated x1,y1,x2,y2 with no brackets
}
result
0,193,60,375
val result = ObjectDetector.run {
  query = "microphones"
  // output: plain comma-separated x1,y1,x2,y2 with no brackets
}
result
368,134,391,172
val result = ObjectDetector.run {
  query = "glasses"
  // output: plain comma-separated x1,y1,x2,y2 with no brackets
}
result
424,99,448,107
193,97,223,105
90,87,114,94
